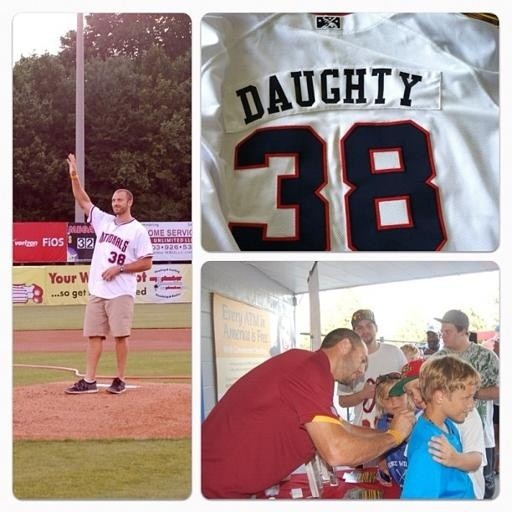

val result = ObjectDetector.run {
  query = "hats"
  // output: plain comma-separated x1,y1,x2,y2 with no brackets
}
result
351,310,375,327
433,310,469,328
389,360,426,396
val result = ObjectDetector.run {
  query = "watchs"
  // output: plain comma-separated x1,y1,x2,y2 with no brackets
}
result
119,264,125,273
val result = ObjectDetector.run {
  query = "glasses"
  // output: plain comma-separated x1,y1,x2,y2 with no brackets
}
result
375,372,400,384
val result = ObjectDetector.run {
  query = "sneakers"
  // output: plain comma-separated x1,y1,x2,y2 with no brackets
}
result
65,380,97,393
105,377,126,393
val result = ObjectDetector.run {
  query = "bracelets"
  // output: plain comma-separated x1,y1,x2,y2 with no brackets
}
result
69,174,79,181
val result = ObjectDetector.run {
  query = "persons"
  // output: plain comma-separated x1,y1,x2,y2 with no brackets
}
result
59,151,153,397
337,306,499,499
200,327,417,499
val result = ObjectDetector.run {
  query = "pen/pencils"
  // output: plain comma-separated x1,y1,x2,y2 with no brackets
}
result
357,472,376,484
358,488,383,501
